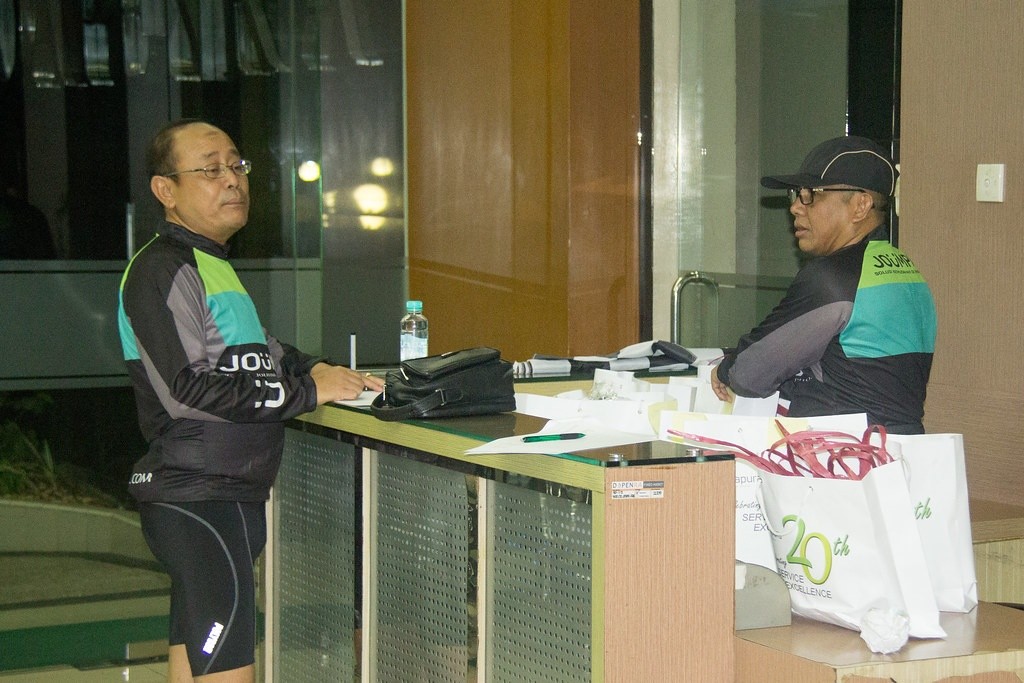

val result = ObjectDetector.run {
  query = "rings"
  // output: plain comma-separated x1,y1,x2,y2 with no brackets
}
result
366,372,372,377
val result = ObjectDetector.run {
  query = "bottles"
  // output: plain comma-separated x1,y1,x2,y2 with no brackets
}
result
399,300,429,361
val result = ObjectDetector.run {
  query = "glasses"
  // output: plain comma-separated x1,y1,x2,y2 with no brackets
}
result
163,159,253,180
787,187,878,209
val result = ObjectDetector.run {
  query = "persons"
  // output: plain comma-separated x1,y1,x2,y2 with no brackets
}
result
116,118,386,683
712,135,938,435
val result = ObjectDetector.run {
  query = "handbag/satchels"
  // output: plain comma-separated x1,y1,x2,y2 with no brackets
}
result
370,347,516,421
568,368,982,654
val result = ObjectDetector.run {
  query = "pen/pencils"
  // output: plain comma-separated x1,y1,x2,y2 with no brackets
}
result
522,433,587,443
350,331,356,371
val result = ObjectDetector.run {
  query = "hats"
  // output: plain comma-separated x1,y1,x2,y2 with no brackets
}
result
760,135,898,196
